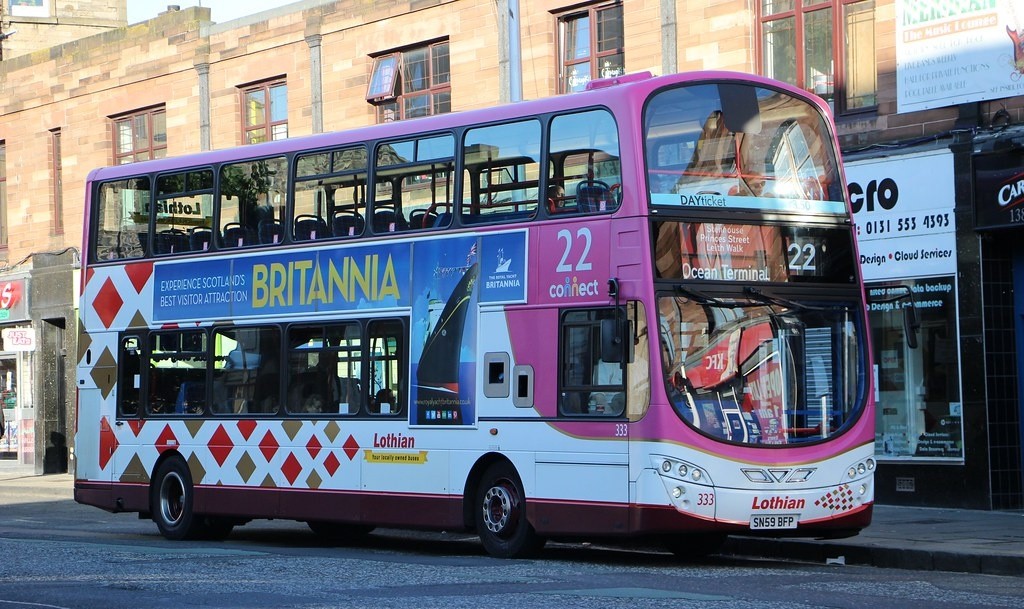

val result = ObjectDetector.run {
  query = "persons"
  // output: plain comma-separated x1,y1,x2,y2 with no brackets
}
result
548,186,565,208
308,349,342,413
374,389,396,413
588,353,650,414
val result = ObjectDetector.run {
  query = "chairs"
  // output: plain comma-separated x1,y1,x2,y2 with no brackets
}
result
119,178,621,415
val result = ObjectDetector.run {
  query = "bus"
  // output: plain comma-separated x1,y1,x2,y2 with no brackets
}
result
73,72,881,564
